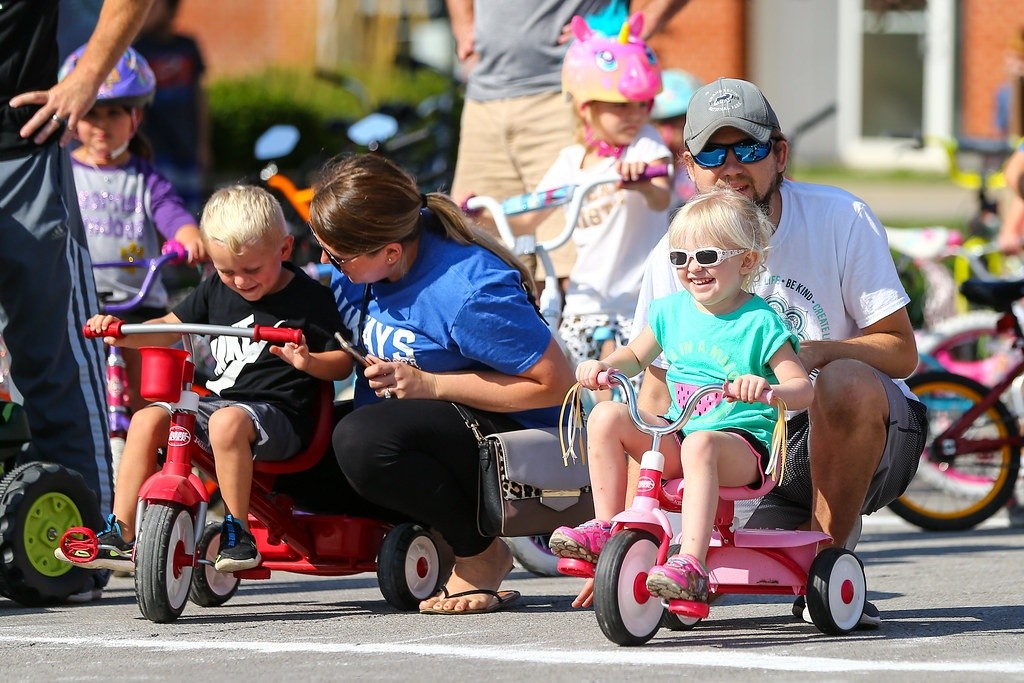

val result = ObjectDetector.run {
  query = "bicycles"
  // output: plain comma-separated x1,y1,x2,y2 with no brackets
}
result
882,275,1024,533
464,163,678,578
928,136,1024,313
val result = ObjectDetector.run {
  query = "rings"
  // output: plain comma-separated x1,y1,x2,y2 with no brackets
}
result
52,114,66,124
385,387,392,399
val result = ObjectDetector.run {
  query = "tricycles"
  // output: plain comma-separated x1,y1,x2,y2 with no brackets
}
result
61,322,442,623
580,368,866,649
0,386,110,610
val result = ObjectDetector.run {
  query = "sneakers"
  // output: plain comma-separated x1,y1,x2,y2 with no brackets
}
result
55,514,135,572
68,576,103,603
792,594,881,625
646,554,709,602
215,514,261,572
549,519,612,564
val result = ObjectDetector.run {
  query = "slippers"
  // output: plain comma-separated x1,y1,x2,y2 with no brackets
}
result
419,586,520,615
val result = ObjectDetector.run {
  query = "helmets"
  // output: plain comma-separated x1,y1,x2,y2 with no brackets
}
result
57,41,156,109
561,11,664,109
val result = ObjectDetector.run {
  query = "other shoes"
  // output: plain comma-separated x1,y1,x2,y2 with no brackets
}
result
1007,503,1024,531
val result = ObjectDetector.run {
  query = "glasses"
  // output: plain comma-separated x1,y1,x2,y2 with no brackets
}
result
306,221,388,274
668,246,748,268
691,138,772,168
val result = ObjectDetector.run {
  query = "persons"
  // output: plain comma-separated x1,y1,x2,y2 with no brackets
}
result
572,77,930,627
955,137,1024,537
549,185,815,601
0,0,205,602
445,0,706,405
55,184,354,574
308,152,579,615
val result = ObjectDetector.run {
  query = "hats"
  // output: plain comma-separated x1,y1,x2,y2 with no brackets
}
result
683,77,781,156
